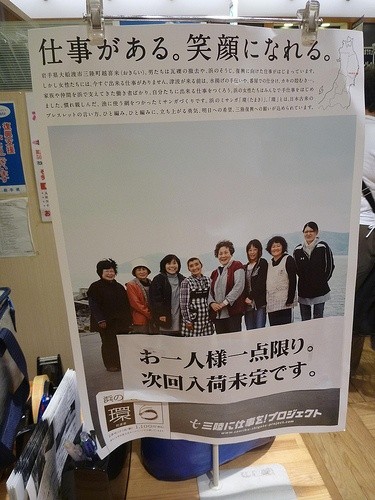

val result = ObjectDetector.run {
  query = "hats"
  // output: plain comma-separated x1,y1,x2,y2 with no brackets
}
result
130,263,152,278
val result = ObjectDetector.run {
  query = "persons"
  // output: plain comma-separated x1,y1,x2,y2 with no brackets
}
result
350,67,375,377
87,258,130,372
126,221,335,338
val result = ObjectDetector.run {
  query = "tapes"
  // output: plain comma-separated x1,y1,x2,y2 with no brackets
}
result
32,375,50,424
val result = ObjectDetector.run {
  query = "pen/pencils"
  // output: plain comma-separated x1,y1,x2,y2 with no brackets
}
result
79,431,101,462
59,438,79,469
